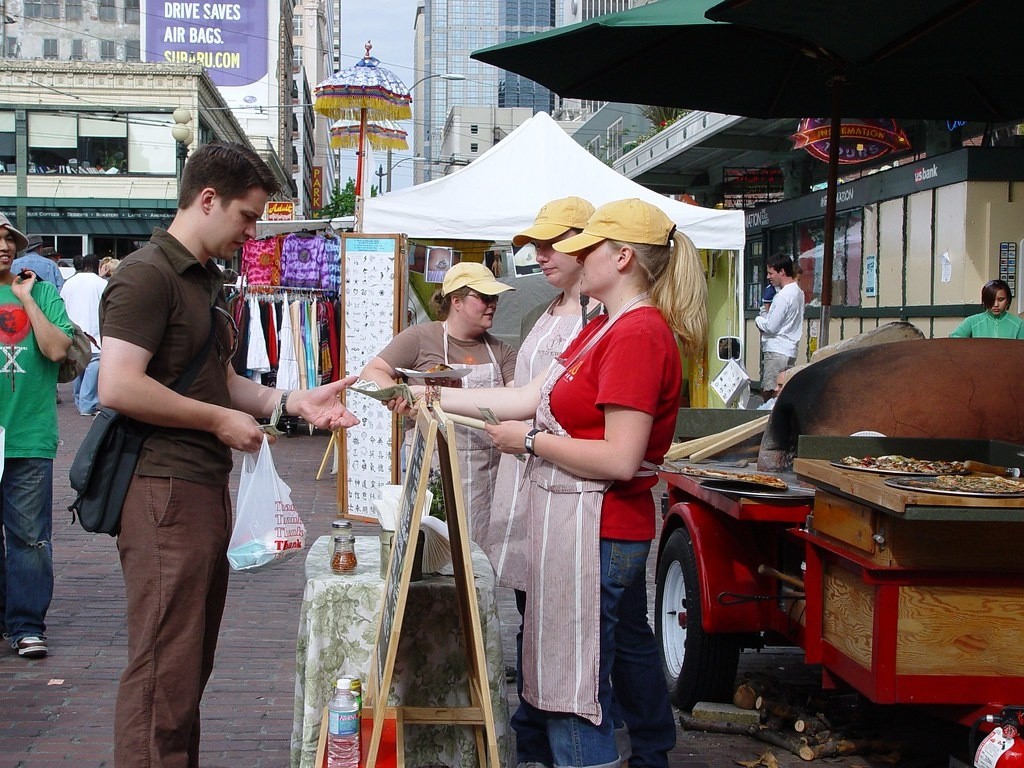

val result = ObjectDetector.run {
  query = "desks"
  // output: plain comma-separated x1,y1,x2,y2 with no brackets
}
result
289,534,510,768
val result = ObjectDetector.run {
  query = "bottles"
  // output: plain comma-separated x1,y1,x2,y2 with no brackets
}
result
328,520,358,575
328,679,360,768
809,321,818,352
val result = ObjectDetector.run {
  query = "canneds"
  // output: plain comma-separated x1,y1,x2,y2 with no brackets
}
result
334,674,362,737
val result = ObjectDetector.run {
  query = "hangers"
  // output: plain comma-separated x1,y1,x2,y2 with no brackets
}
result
254,225,275,241
229,285,338,302
325,222,335,234
296,223,314,239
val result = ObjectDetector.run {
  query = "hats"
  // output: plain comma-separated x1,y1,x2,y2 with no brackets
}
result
40,247,58,257
0,212,29,253
440,261,517,295
552,198,677,257
511,197,596,247
26,234,42,251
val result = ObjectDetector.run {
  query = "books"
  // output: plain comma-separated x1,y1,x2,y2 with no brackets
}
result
710,357,751,407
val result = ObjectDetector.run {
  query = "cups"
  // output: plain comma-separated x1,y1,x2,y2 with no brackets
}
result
380,530,395,580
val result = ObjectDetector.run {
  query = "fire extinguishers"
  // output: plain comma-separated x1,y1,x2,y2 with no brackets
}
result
967,702,1024,768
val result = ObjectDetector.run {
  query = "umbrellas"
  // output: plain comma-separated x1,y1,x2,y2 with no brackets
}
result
470,0,1023,339
313,66,412,194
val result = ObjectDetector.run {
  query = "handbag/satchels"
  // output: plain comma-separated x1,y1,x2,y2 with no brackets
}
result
17,269,102,384
226,435,306,575
67,404,151,537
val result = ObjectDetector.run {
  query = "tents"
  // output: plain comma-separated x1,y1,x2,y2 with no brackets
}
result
360,110,749,407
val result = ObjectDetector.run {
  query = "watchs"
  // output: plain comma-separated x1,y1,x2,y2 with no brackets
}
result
524,429,541,458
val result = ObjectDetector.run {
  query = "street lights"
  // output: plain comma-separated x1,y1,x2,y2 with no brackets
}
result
385,74,466,193
172,108,194,183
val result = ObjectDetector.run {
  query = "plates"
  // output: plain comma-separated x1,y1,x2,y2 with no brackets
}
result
395,367,473,380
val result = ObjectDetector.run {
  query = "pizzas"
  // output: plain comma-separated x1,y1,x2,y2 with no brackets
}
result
841,454,967,475
897,475,1024,494
699,468,787,488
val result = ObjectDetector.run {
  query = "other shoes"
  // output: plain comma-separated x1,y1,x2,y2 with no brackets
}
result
18,636,48,659
81,410,100,416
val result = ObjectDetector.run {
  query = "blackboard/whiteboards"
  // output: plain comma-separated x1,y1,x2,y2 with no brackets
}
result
371,397,493,719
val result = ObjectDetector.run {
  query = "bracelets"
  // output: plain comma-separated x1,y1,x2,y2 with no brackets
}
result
425,384,441,411
280,391,292,415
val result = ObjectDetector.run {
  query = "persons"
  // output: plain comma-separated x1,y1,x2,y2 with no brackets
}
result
755,252,805,401
360,262,516,681
0,212,76,657
485,196,631,768
98,142,359,768
948,279,1024,340
10,235,123,416
381,198,708,767
